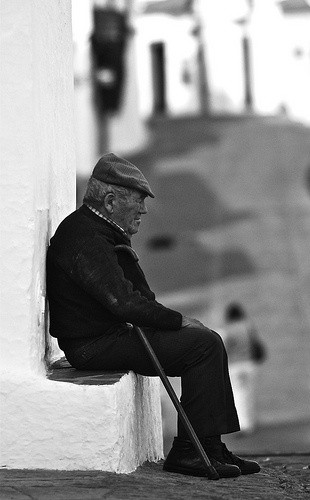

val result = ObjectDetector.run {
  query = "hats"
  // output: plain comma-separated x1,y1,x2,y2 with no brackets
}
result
93,153,155,199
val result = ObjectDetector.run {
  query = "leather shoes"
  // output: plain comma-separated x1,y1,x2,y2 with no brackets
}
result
205,440,260,474
163,437,241,477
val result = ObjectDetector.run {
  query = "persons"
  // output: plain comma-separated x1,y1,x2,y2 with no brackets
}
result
44,152,262,478
